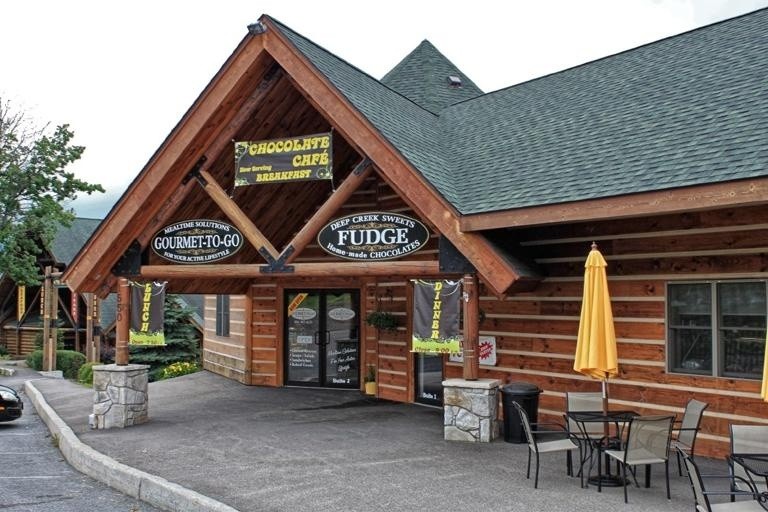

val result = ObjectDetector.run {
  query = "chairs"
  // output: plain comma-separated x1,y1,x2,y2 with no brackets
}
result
728,423,768,502
597,412,677,504
675,445,768,512
566,391,619,480
648,397,710,488
512,400,584,489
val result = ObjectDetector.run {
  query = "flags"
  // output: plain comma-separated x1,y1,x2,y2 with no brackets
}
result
408,276,464,354
127,280,170,347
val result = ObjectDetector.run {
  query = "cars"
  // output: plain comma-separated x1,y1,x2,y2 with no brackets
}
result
0,383,23,421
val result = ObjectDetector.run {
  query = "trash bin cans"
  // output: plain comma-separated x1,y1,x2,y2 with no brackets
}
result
498,383,543,444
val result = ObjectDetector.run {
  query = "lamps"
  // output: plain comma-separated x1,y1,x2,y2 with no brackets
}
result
246,21,268,36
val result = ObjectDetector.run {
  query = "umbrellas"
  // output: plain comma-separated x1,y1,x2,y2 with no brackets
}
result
572,240,621,475
